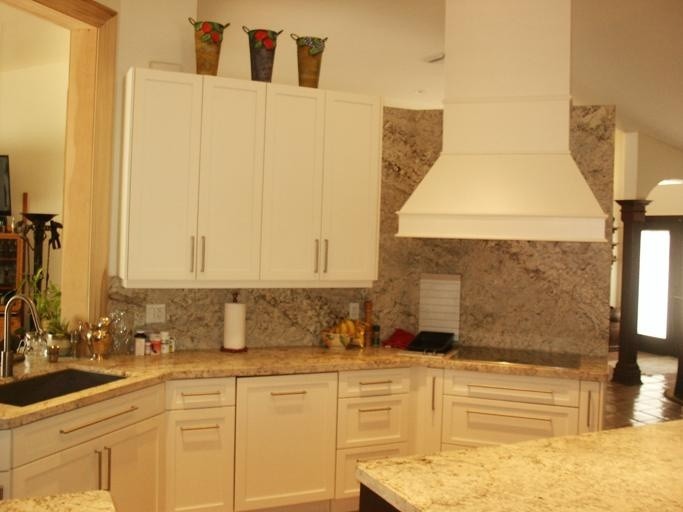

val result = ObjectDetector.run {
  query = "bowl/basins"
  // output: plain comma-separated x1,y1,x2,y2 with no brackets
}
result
321,331,351,351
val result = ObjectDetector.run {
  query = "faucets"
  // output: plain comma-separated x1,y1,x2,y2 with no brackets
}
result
1,291,47,378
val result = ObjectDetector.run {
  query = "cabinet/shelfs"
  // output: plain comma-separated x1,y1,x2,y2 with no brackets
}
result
0,0,118,358
260,82,384,290
1,380,166,512
335,367,417,501
235,371,338,511
442,369,605,453
108,67,266,289
167,376,236,512
415,366,445,454
0,232,24,351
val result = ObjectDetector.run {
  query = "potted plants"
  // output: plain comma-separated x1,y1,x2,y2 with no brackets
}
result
35,282,72,357
19,267,51,331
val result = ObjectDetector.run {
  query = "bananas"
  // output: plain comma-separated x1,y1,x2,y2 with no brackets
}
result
334,317,356,336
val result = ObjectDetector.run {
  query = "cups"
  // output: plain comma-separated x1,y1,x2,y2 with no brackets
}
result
23,332,60,362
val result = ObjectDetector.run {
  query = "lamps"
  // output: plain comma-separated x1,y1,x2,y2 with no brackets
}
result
146,302,166,325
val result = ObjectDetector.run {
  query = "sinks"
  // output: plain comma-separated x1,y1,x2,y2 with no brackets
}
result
0,362,135,409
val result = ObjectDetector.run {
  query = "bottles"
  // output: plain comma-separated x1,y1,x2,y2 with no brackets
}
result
133,329,176,356
69,310,130,361
6,217,16,232
372,314,380,346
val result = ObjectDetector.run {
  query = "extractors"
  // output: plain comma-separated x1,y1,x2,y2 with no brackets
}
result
391,0,611,246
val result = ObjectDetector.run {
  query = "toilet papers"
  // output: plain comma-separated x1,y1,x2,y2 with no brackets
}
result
222,299,246,353
